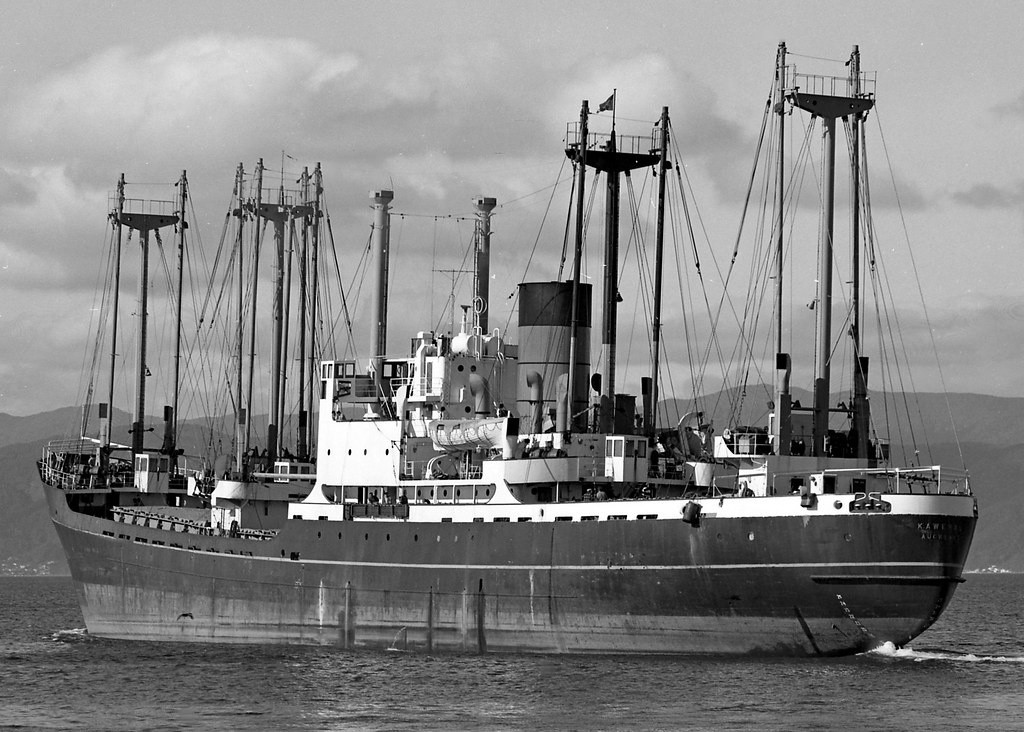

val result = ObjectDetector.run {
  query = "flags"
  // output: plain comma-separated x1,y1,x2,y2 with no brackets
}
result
600,93,614,111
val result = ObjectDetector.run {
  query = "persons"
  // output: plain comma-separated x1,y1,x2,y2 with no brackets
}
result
597,486,607,501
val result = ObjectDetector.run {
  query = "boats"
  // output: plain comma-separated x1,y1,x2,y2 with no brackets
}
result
33,39,980,656
